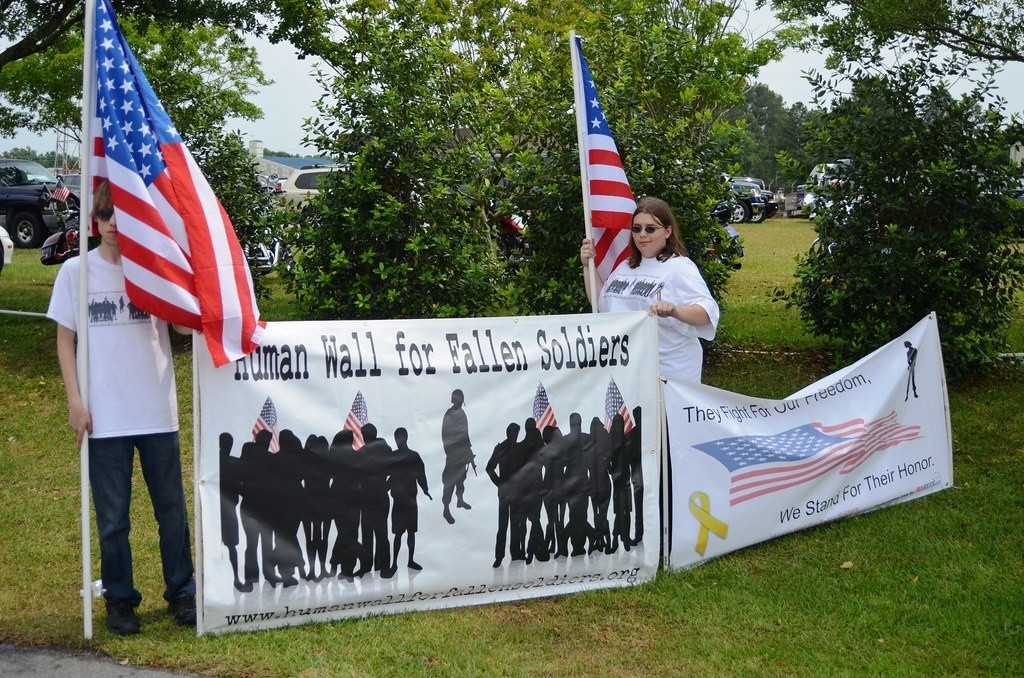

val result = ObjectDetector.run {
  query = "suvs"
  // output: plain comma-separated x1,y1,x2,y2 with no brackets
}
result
277,164,348,208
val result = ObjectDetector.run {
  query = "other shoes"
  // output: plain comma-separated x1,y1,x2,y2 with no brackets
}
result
105,600,140,635
168,595,197,626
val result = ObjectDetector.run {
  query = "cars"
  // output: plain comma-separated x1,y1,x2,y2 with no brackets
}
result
0,159,81,249
711,173,778,225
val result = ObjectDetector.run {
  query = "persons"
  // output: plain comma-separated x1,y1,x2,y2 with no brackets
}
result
581,197,719,556
46,180,198,637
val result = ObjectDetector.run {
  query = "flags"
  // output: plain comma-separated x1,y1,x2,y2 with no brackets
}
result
38,183,53,208
80,0,267,368
252,396,280,453
51,177,69,202
569,31,637,285
604,377,636,436
533,382,557,436
343,390,370,450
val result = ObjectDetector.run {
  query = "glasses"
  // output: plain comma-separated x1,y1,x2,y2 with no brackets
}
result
631,226,663,233
93,207,113,222
